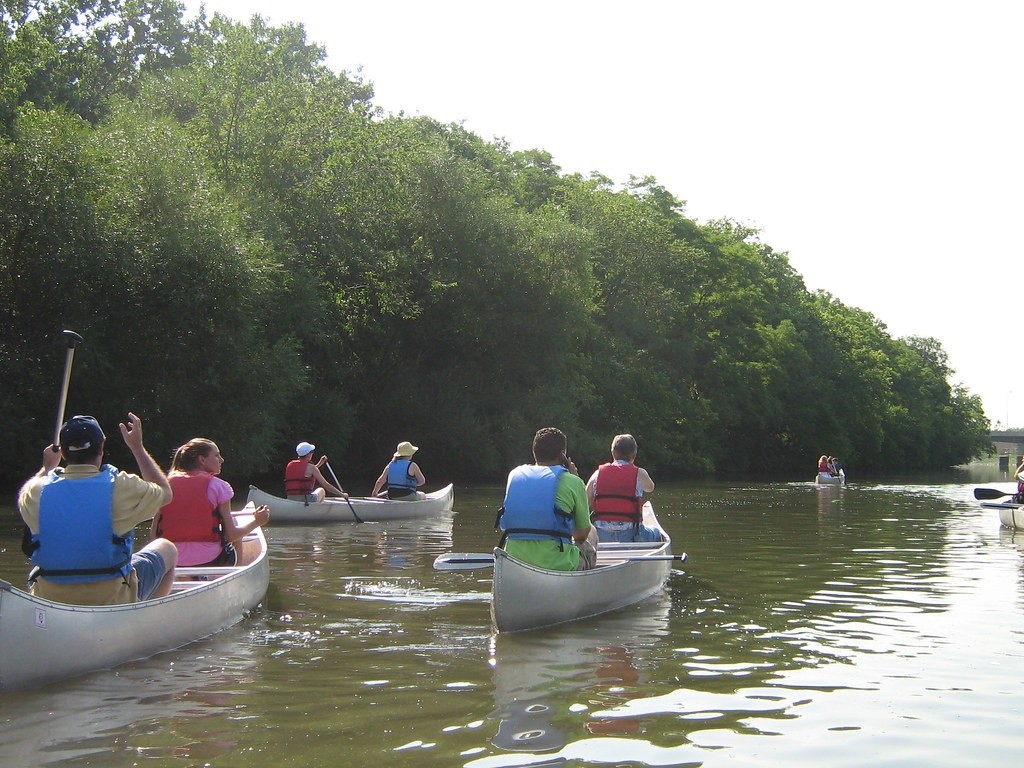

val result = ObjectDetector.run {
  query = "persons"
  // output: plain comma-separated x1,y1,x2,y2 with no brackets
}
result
150,437,270,582
498,425,599,571
284,441,348,502
371,441,427,501
1013,455,1024,504
18,409,179,607
817,455,847,478
587,434,667,550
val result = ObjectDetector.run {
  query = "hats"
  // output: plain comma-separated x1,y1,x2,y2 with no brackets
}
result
393,442,418,457
296,442,315,456
60,415,106,451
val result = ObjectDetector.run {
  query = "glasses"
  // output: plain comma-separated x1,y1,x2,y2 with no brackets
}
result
311,450,314,454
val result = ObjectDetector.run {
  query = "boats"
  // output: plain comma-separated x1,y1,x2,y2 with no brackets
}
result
246,482,454,523
492,500,675,633
814,468,846,485
998,499,1024,529
0,501,270,694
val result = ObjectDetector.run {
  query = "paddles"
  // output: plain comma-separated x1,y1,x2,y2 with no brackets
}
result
432,552,688,571
830,456,844,486
973,488,1018,500
377,490,388,498
21,329,85,558
322,456,365,523
230,504,264,518
